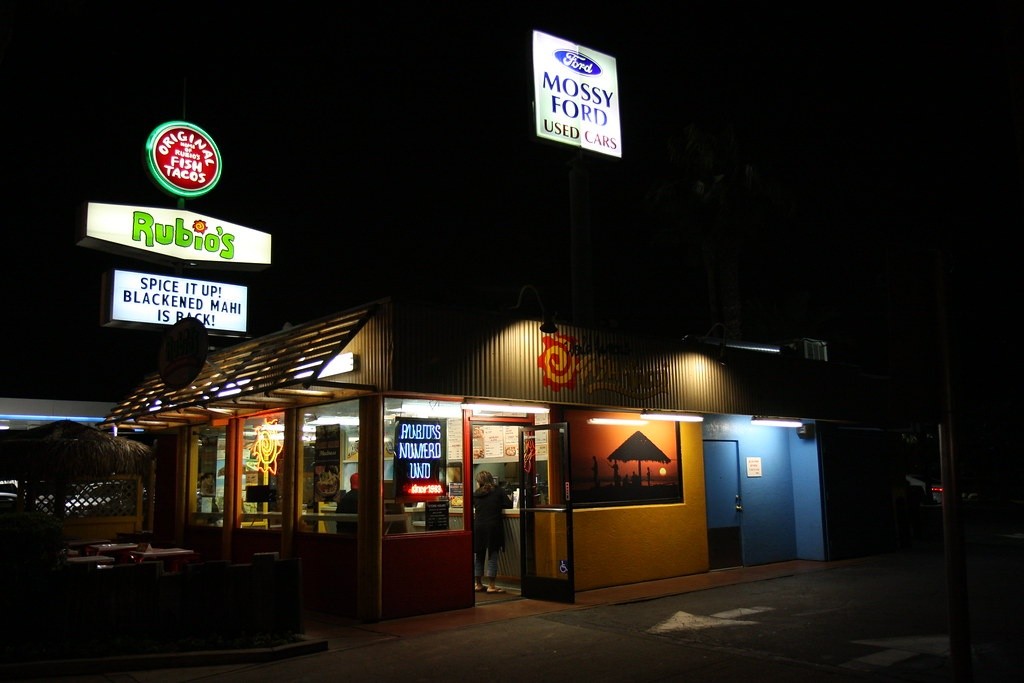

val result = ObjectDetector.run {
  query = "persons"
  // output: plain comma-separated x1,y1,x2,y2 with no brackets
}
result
334,474,359,532
472,471,513,594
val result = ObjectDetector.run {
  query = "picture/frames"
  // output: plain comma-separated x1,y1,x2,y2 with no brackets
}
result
561,407,684,509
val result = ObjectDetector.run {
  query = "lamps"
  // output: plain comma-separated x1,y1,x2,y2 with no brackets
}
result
460,397,550,414
751,417,803,428
126,352,354,411
640,411,704,421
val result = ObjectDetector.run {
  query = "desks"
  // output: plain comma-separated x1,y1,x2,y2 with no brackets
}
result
307,511,411,535
64,531,198,572
193,509,283,527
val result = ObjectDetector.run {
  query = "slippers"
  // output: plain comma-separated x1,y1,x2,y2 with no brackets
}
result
475,585,488,592
486,587,507,595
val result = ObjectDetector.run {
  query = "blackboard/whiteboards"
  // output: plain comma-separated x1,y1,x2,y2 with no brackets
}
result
425,501,449,531
